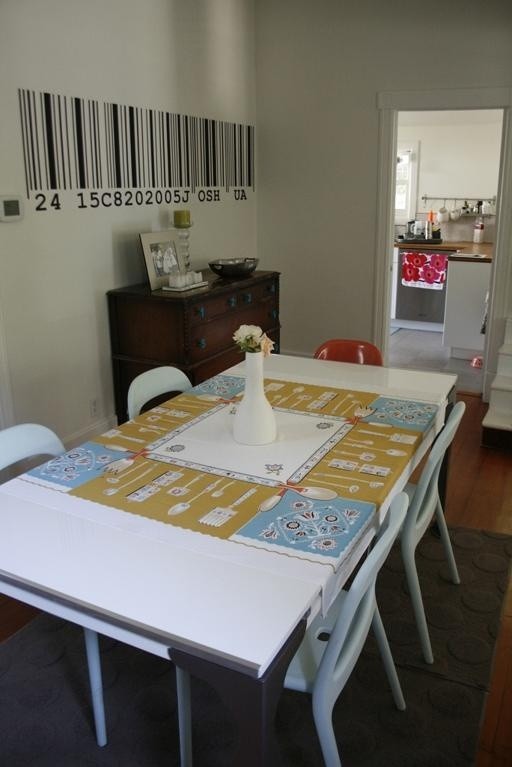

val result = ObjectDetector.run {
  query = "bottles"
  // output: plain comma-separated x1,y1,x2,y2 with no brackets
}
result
472,217,484,242
424,221,432,239
167,268,199,288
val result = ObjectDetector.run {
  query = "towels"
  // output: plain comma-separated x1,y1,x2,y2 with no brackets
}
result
400,250,448,290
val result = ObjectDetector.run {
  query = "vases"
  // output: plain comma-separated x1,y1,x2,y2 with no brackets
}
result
232,349,274,446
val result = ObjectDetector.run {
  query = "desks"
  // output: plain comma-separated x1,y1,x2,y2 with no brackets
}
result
0,352,460,767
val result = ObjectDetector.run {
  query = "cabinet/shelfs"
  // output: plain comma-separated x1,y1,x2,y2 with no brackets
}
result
106,269,282,428
395,248,455,325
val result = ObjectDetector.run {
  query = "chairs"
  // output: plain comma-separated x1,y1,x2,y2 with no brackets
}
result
0,424,109,749
315,338,384,367
127,365,193,420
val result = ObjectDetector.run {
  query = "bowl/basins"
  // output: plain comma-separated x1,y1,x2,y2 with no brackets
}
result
209,257,258,276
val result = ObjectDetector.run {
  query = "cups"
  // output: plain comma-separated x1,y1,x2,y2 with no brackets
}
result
397,234,404,242
410,220,423,234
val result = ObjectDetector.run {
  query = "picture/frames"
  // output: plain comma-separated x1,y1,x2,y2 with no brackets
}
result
141,229,185,292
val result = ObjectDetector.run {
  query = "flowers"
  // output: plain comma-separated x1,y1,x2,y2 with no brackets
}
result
232,323,274,359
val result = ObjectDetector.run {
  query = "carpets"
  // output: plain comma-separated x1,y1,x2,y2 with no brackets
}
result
1,522,511,766
